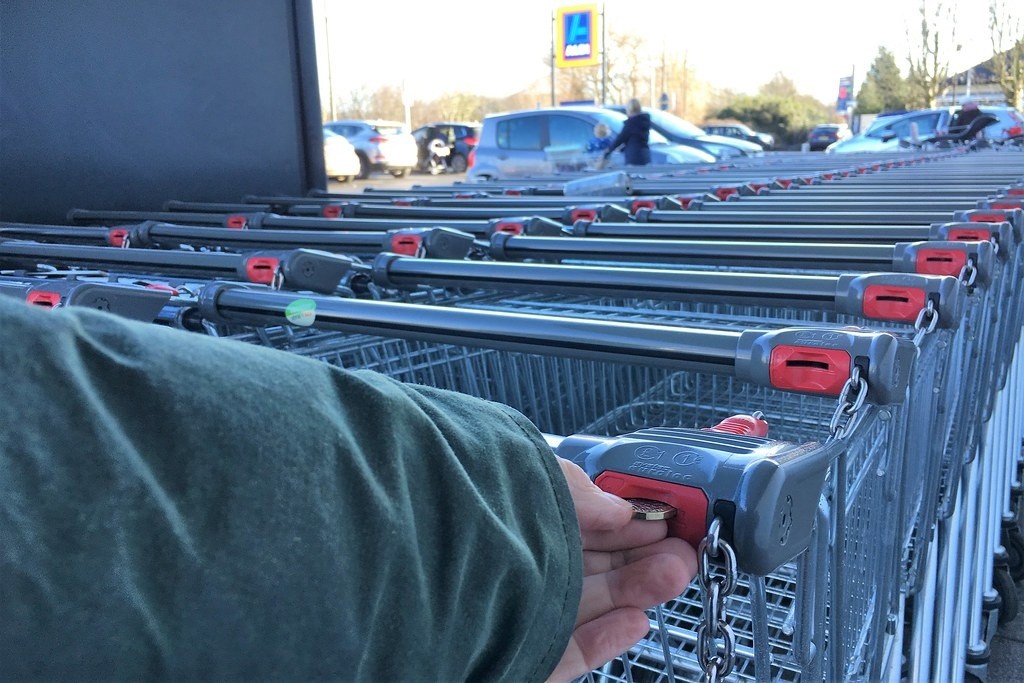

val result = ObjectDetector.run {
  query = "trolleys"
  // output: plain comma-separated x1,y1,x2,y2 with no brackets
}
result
0,149,1024,683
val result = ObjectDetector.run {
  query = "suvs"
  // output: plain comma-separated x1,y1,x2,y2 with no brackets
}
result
809,124,844,151
603,106,764,160
412,123,484,174
702,124,772,147
323,120,418,180
467,107,716,191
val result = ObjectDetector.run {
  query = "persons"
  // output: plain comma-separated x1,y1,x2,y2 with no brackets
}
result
586,124,611,153
0,293,698,683
606,99,651,165
952,101,979,143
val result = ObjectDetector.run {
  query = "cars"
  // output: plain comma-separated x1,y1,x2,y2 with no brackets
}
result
825,106,1024,155
322,129,360,182
